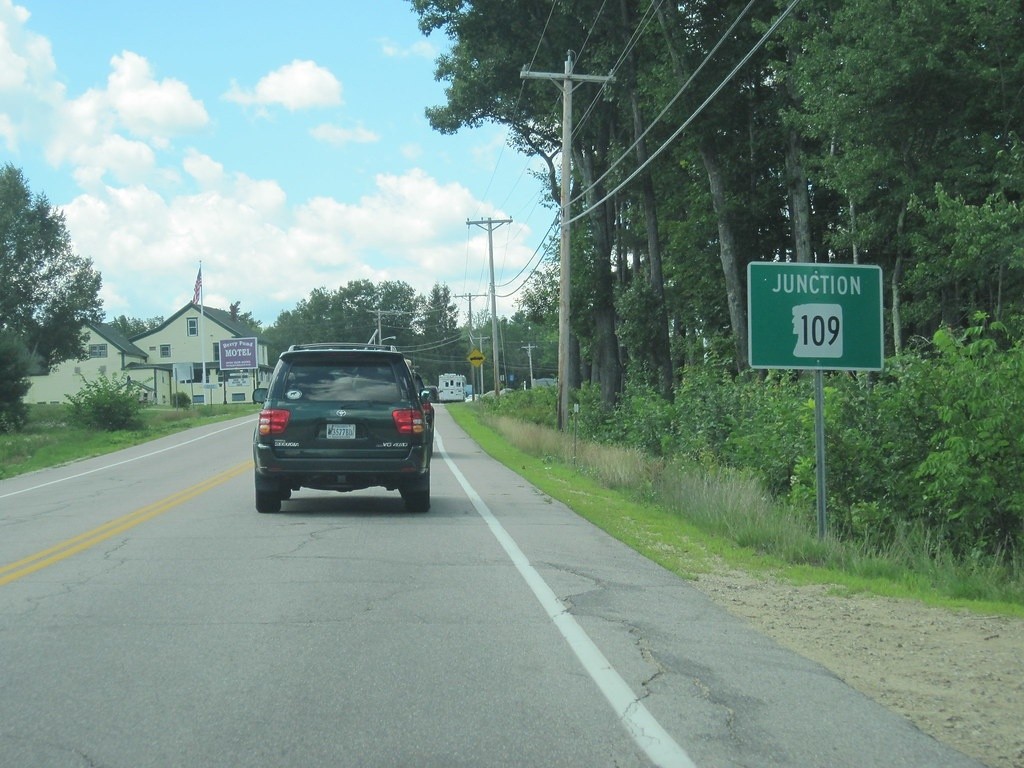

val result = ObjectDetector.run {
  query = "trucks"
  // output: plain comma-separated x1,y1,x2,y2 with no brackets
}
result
438,373,467,403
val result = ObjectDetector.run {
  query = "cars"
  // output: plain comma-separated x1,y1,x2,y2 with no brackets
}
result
409,371,438,459
252,343,430,513
465,395,478,403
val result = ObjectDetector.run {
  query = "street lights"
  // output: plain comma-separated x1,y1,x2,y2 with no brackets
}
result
379,336,397,346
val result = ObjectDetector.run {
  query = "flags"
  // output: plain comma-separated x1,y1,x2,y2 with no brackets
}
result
193,268,202,304
